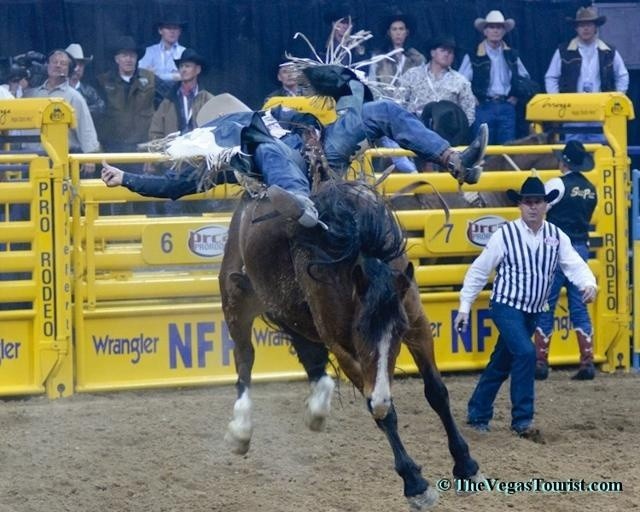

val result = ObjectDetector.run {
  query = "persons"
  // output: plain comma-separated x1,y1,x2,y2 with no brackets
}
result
545,7,630,144
0,11,488,228
453,180,598,437
458,10,531,160
534,139,598,379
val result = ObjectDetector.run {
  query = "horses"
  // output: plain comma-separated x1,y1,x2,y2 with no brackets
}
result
217,150,489,510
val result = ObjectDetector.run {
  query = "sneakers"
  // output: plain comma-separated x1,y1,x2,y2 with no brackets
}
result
471,422,492,433
509,424,547,445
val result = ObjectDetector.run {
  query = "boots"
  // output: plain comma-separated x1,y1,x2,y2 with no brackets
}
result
571,328,595,380
534,326,553,380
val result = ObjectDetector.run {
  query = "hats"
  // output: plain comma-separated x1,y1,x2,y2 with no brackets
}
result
507,177,559,204
64,11,254,128
552,139,594,171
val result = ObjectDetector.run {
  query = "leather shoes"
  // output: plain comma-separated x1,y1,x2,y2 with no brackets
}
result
267,184,319,229
447,123,489,185
